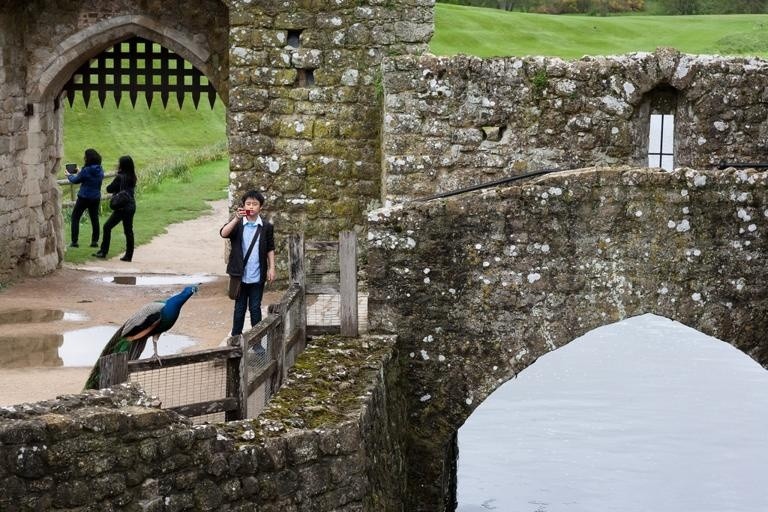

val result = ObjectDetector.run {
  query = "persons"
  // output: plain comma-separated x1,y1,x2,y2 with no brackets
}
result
64,148,104,247
220,190,277,356
96,156,137,261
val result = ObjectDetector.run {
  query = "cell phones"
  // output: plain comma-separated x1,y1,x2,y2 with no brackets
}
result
243,209,255,216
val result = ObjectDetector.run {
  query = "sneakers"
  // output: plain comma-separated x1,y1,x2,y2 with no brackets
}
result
120,252,134,263
89,241,99,248
66,242,79,248
252,345,265,356
91,250,107,258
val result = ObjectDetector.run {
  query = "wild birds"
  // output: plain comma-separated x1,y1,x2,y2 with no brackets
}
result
83,282,202,391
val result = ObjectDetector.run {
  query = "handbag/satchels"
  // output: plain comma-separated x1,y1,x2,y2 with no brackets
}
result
109,190,131,210
227,276,241,300
